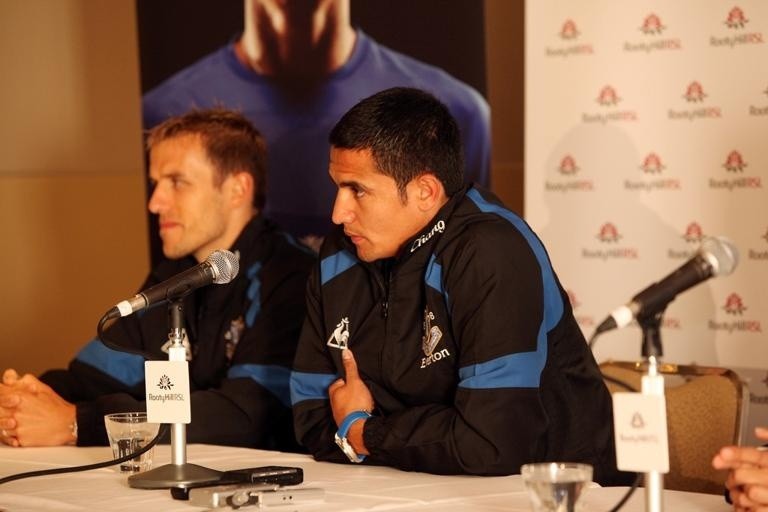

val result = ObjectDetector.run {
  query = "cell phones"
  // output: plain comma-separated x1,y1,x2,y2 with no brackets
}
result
220,462,305,488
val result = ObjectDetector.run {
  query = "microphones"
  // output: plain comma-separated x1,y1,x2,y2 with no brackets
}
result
108,248,241,330
596,237,740,338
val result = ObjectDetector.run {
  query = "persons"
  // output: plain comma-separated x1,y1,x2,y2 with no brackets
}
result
141,2,497,248
1,99,323,456
287,85,646,493
710,426,768,512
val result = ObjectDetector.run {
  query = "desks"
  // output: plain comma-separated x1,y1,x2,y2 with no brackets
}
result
1,438,733,512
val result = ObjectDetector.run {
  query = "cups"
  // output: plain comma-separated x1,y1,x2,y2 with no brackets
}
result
104,412,161,475
520,461,594,512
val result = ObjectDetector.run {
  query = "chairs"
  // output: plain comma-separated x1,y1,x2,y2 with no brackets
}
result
594,359,751,492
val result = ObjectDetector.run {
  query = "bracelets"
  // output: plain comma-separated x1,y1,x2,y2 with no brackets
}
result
70,418,77,438
333,410,375,464
